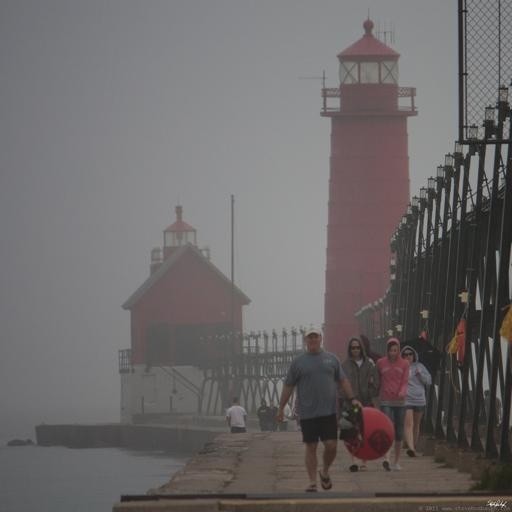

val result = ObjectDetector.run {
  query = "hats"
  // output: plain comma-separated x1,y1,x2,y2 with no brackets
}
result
305,325,320,337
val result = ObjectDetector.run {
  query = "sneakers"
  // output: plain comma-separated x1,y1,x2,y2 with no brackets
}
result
383,460,391,471
406,449,415,457
360,464,368,471
393,464,400,470
350,464,358,472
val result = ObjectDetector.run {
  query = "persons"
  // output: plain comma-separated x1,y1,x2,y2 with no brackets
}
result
337,335,381,471
374,336,410,472
226,398,300,434
483,389,502,428
275,325,364,493
400,344,433,456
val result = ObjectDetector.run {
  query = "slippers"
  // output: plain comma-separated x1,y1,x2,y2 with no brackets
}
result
306,484,317,492
319,469,332,489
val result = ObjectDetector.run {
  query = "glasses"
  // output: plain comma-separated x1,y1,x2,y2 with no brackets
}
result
404,353,413,356
351,346,360,350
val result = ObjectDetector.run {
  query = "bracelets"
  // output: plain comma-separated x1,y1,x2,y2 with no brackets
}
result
349,397,357,402
